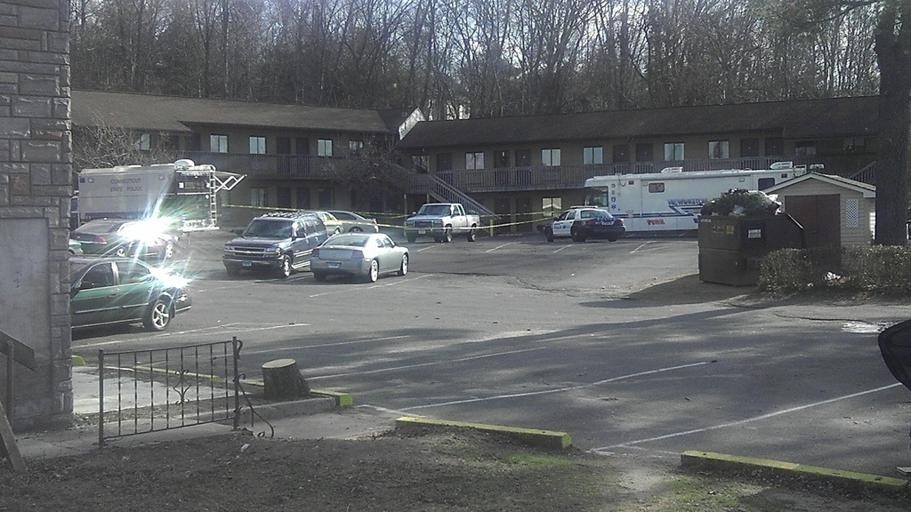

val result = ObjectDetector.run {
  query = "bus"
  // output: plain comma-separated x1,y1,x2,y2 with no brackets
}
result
582,161,807,234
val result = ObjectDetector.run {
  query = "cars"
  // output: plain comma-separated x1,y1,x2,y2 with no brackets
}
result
544,204,625,244
222,207,409,283
70,216,192,333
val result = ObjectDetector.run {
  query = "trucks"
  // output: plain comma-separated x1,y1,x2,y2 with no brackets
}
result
71,158,247,235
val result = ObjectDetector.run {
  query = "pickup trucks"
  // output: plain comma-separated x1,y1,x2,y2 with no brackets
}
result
404,203,480,242
